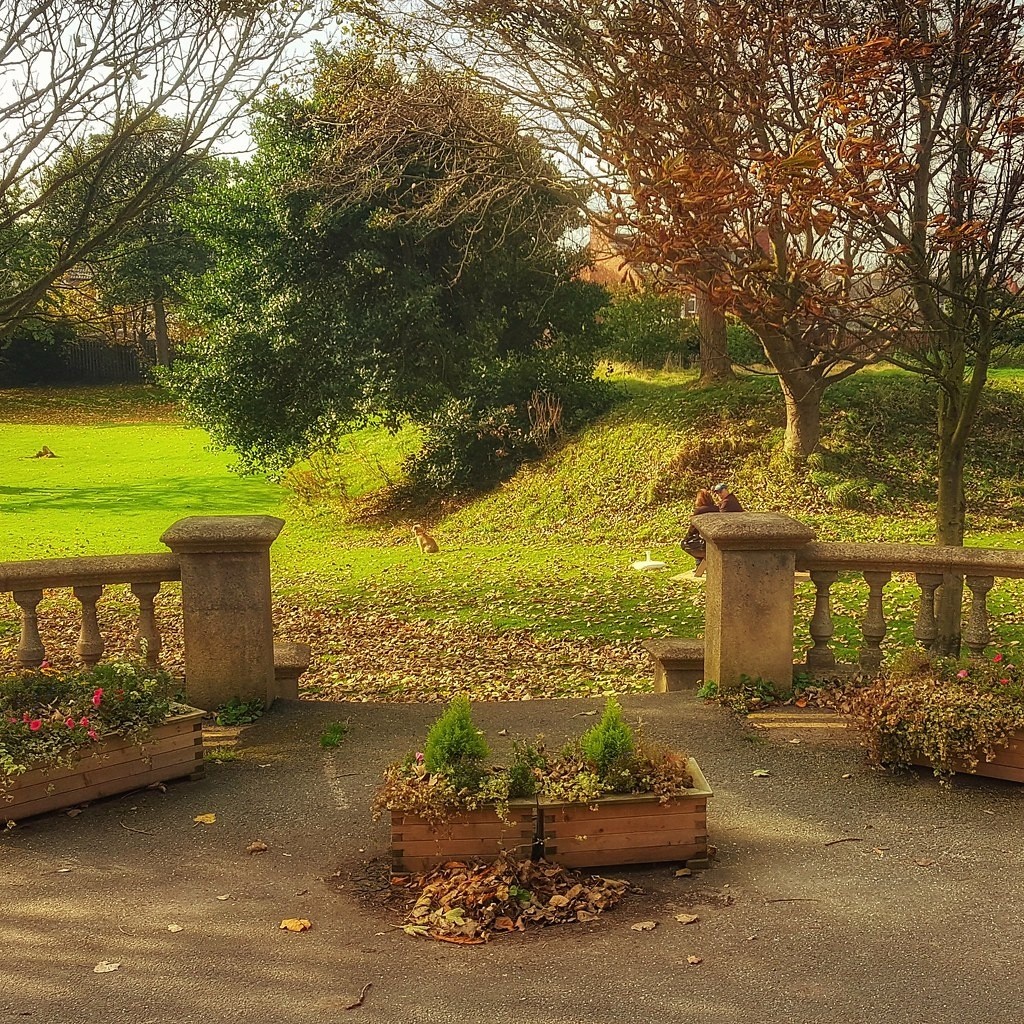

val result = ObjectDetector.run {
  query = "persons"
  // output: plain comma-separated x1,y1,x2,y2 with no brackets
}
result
680,489,719,573
715,483,744,512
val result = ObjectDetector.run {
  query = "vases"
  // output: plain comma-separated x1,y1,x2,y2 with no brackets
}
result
864,721,1024,784
0,702,209,826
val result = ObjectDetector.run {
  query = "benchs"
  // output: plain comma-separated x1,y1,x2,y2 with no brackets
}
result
685,546,707,578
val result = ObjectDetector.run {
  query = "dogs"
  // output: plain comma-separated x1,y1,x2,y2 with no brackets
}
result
411,524,440,553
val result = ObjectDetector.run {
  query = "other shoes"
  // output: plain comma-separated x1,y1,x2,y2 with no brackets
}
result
692,566,698,572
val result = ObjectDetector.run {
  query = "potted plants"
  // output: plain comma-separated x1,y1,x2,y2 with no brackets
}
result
537,694,713,870
385,693,538,882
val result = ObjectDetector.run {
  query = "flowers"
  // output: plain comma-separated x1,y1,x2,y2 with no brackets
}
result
934,645,1024,706
0,637,177,803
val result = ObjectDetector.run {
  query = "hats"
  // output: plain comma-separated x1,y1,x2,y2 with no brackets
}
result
714,483,726,493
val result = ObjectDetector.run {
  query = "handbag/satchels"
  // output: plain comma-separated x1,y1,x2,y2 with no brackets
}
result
681,533,706,550
